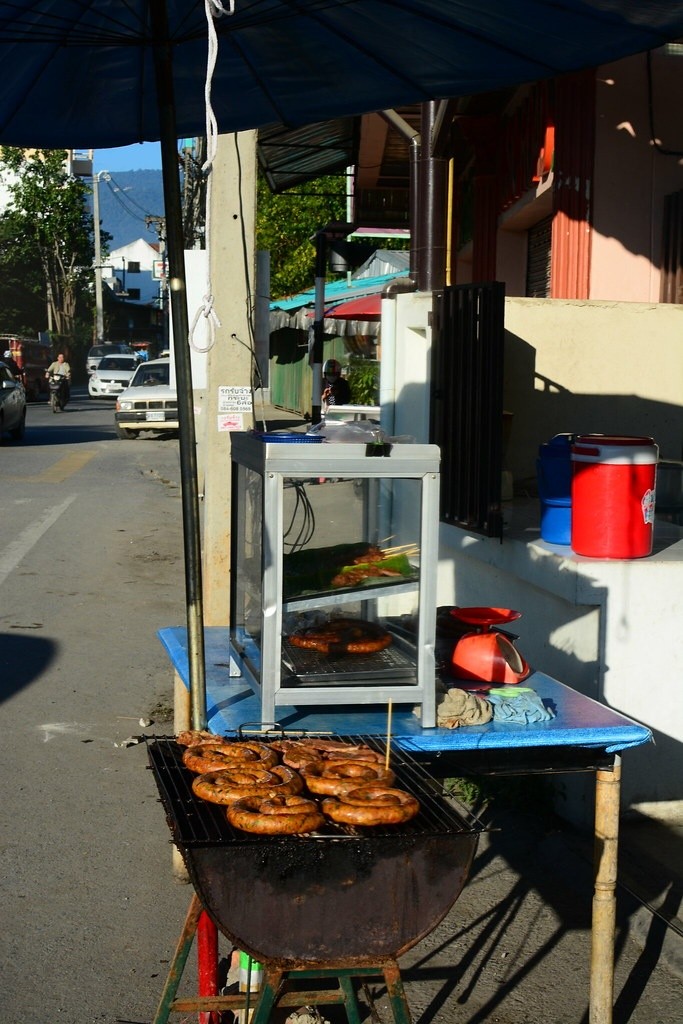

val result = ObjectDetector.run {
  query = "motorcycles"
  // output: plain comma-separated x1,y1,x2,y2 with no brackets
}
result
44,368,72,413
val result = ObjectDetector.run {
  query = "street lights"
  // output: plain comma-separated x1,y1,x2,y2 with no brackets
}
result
94,170,111,344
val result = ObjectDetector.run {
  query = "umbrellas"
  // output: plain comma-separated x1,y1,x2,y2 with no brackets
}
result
0,0,683,737
304,294,381,322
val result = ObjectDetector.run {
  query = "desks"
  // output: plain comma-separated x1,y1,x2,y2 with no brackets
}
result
158,626,655,1024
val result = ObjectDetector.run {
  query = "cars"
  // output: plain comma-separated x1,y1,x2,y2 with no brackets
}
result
86,344,134,375
88,354,138,400
0,361,26,439
115,357,179,440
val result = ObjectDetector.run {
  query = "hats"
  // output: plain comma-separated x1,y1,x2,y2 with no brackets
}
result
324,358,341,376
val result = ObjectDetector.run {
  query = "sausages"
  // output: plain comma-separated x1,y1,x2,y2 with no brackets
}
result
183,739,418,833
290,617,393,652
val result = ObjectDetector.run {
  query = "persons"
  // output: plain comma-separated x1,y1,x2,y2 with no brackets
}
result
45,354,71,409
3,351,24,380
112,361,117,367
144,374,160,384
311,359,352,405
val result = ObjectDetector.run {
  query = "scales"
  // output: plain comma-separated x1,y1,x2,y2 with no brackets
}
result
448,604,530,685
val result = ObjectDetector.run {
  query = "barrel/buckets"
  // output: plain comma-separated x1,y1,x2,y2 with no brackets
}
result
535,433,579,545
571,433,659,560
